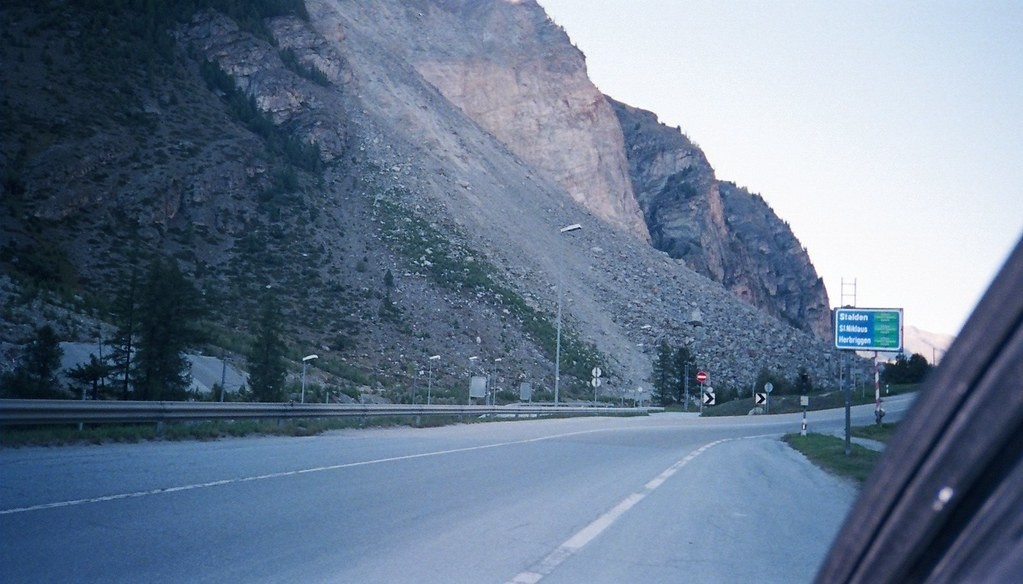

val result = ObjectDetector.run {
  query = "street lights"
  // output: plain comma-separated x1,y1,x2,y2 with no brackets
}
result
427,355,441,405
554,224,582,411
468,356,478,406
301,354,319,404
493,358,503,406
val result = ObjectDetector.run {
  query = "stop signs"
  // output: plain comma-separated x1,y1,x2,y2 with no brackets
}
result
696,371,707,382
